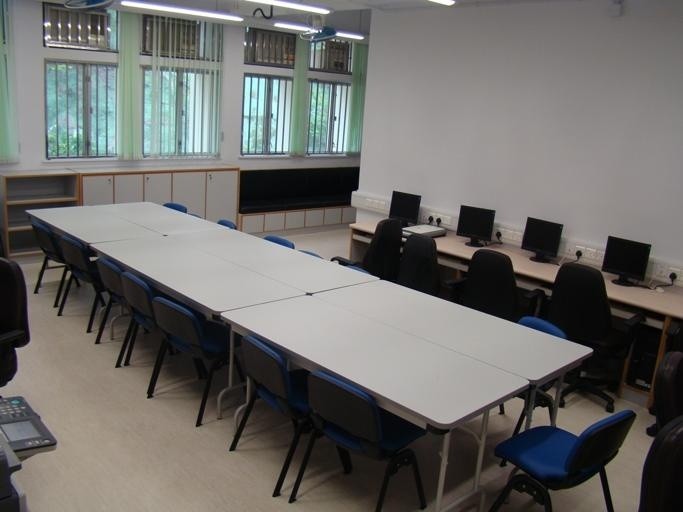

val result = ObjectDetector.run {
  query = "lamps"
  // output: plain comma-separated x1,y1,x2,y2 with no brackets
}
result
122,0,245,27
245,1,335,17
273,19,367,43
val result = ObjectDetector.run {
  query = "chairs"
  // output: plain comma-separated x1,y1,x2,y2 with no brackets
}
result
0,258,30,389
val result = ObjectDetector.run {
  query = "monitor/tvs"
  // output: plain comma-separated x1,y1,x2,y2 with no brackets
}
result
601,235,652,286
456,204,496,247
520,216,563,262
388,190,422,227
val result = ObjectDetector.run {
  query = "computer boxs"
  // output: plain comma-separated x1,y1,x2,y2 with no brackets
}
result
625,325,663,393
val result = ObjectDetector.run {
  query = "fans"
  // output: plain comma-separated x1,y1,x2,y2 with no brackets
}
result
63,0,115,12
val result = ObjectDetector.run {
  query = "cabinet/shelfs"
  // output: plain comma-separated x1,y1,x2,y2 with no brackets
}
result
0,168,80,258
69,163,240,225
